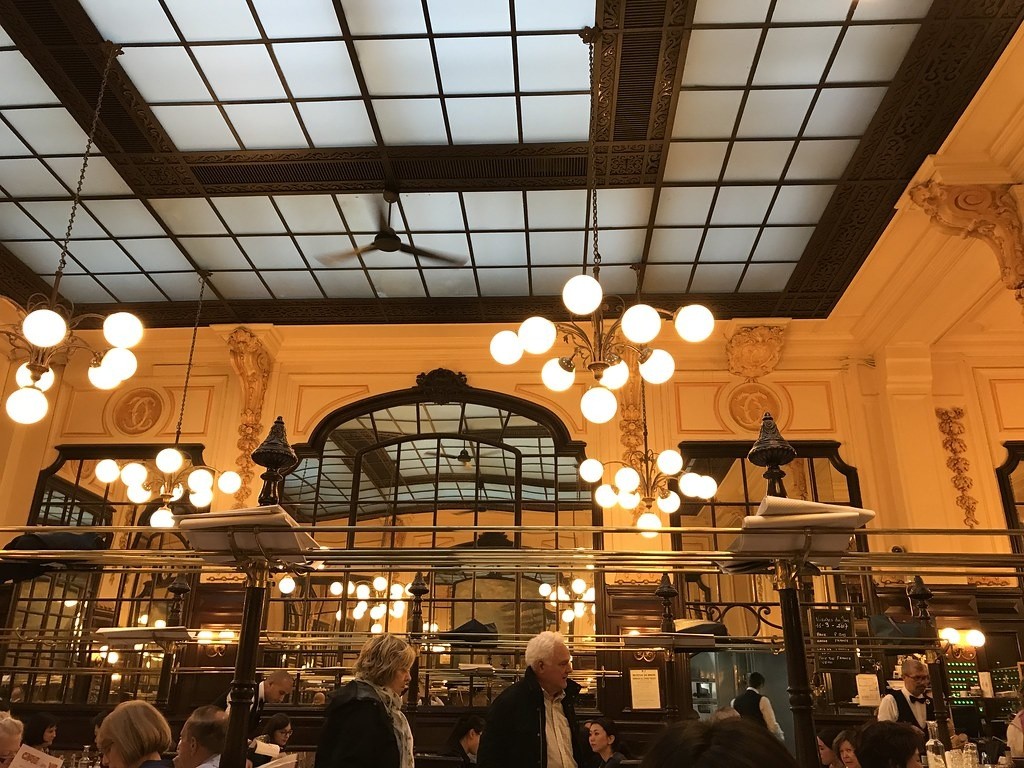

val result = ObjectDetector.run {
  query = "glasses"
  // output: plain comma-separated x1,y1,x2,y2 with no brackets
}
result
274,729,293,736
904,673,929,681
100,739,115,757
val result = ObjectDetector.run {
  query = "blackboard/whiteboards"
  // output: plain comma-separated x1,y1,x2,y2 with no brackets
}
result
808,608,860,674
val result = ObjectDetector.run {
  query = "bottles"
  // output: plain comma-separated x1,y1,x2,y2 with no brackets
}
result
949,742,979,768
41,747,63,760
925,721,947,768
78,745,102,768
68,754,76,768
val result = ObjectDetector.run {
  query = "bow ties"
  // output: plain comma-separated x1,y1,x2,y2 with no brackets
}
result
910,694,926,704
258,698,264,708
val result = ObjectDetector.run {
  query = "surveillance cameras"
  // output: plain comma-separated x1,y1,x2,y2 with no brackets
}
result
890,545,904,554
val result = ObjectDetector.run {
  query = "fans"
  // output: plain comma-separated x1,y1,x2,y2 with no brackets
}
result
452,481,514,515
314,187,469,266
424,439,501,470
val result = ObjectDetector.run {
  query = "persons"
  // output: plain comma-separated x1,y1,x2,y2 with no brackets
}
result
579,660,1024,768
314,634,416,768
477,631,585,767
441,715,485,768
0,669,325,768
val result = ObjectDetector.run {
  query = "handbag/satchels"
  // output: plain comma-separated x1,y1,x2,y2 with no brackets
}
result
438,618,498,648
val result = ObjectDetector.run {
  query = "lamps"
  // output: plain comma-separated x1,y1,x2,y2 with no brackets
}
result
538,508,596,622
422,620,451,652
941,627,985,661
197,628,235,656
95,645,119,667
278,460,330,594
330,460,414,633
578,265,717,539
95,271,242,528
489,22,714,424
0,40,143,425
